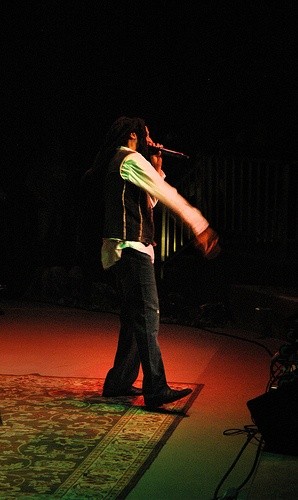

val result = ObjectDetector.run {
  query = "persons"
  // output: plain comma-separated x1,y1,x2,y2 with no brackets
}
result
99,115,218,407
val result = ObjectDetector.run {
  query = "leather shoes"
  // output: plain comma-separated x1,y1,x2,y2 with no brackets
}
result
103,386,143,396
147,386,192,409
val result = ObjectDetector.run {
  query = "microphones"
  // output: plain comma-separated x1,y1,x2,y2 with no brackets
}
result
148,145,189,159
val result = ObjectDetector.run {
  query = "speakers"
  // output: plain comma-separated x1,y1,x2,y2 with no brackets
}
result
247,380,298,456
